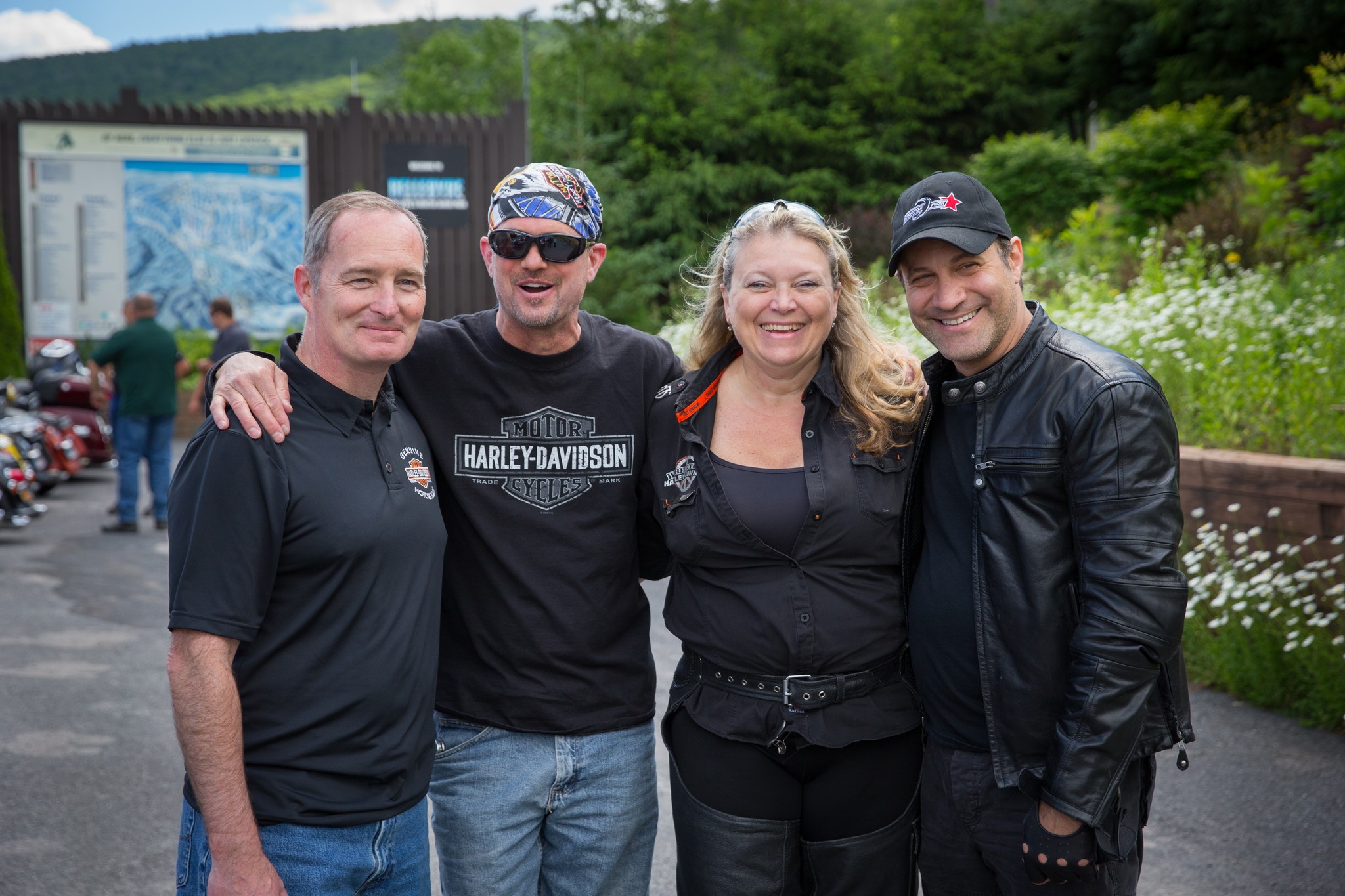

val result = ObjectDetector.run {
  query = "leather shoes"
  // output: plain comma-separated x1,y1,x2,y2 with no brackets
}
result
156,519,169,530
102,521,136,533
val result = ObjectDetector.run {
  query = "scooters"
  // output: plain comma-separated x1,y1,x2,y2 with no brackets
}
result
1,358,117,528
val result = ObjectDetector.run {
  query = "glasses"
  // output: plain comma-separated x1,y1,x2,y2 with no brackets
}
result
487,229,595,262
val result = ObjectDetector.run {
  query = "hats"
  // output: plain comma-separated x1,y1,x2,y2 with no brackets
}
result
886,170,1013,277
486,162,603,243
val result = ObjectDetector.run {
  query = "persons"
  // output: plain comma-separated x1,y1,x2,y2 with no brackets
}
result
162,189,448,896
886,171,1195,896
209,161,686,896
650,199,932,896
86,290,252,533
